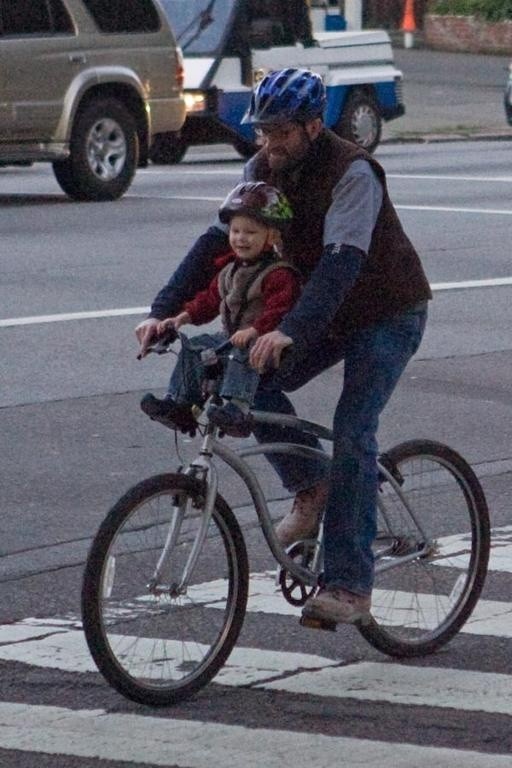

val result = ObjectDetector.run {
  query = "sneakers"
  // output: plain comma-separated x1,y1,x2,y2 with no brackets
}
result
205,399,256,440
301,580,374,623
274,478,331,548
139,390,206,440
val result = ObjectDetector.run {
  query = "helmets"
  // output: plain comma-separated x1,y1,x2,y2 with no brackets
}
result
218,180,296,236
238,65,329,128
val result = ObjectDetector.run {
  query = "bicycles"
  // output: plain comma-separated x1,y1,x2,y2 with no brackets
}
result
81,330,490,706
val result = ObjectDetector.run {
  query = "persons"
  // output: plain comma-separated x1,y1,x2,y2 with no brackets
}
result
126,63,434,629
137,176,305,441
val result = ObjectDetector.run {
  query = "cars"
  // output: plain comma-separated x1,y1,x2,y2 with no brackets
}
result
0,0,188,201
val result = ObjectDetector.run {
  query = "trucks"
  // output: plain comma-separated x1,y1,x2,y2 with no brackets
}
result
149,0,406,167
